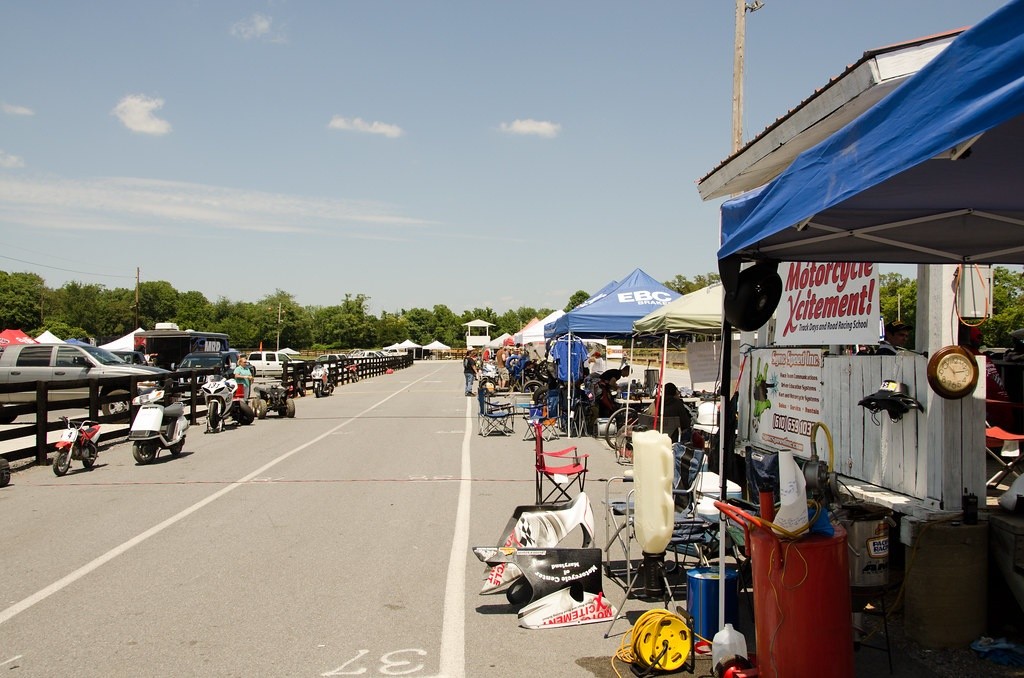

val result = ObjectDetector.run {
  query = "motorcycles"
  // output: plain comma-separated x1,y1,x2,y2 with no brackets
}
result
250,380,296,420
51,415,102,477
310,365,329,398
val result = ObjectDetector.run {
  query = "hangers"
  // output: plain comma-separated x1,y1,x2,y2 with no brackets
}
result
558,332,582,342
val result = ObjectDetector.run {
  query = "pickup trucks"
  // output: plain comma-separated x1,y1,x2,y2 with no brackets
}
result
0,342,177,425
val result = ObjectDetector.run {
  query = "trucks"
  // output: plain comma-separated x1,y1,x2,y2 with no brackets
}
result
133,322,229,377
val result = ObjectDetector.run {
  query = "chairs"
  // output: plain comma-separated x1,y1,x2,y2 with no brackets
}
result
984,420,1024,487
475,385,707,592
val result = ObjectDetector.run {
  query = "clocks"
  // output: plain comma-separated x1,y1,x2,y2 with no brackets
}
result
926,345,979,400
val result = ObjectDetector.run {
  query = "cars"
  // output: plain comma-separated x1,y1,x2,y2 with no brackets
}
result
310,348,401,382
246,350,309,380
109,350,149,366
178,350,241,405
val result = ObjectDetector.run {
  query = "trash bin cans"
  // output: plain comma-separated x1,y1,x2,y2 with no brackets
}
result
688,567,739,643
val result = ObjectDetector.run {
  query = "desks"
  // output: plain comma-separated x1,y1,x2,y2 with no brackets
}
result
614,397,700,423
495,392,535,414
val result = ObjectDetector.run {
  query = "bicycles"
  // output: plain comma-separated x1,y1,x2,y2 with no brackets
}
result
604,401,694,451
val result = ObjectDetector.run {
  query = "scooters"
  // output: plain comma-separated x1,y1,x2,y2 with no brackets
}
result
126,387,191,466
200,374,239,434
